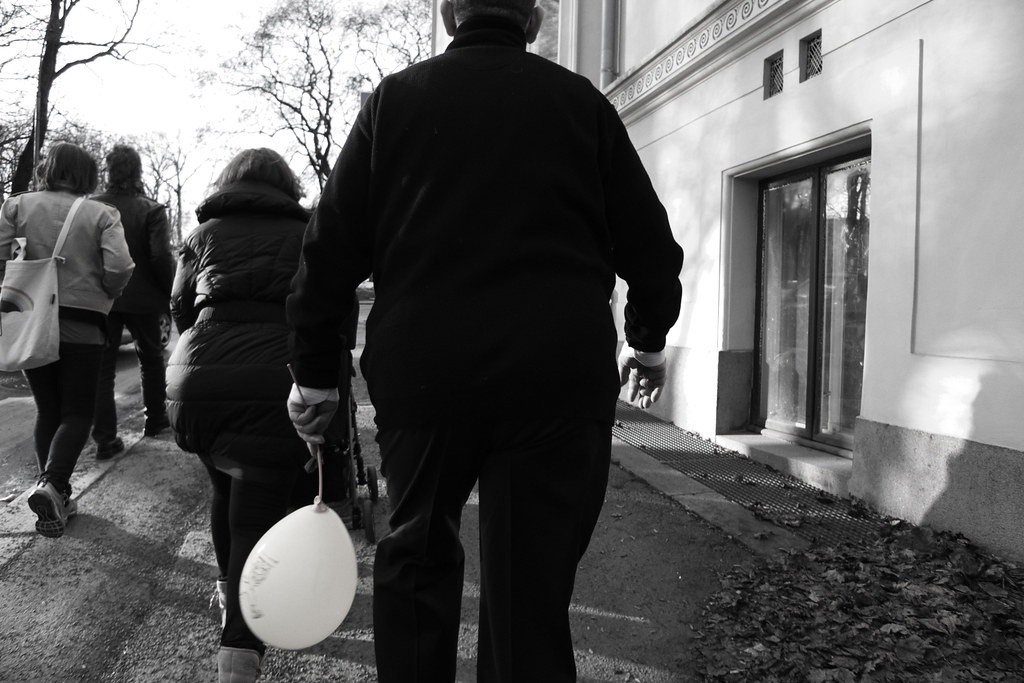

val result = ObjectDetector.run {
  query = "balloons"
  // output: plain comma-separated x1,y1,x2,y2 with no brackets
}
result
239,495,358,650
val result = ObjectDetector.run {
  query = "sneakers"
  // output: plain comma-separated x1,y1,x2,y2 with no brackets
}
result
61,499,77,520
28,480,66,539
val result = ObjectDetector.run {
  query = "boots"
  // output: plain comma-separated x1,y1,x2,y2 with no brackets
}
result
216,579,226,629
218,646,262,683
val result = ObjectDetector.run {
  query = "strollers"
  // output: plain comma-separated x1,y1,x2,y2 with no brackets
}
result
308,383,380,544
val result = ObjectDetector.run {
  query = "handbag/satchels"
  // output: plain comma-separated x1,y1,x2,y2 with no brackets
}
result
0,196,86,373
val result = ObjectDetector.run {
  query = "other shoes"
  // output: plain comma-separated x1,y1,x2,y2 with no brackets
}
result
144,417,170,436
96,437,124,459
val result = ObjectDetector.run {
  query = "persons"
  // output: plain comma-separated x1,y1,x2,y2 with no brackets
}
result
87,145,178,461
163,148,317,683
287,0,685,682
0,143,135,538
